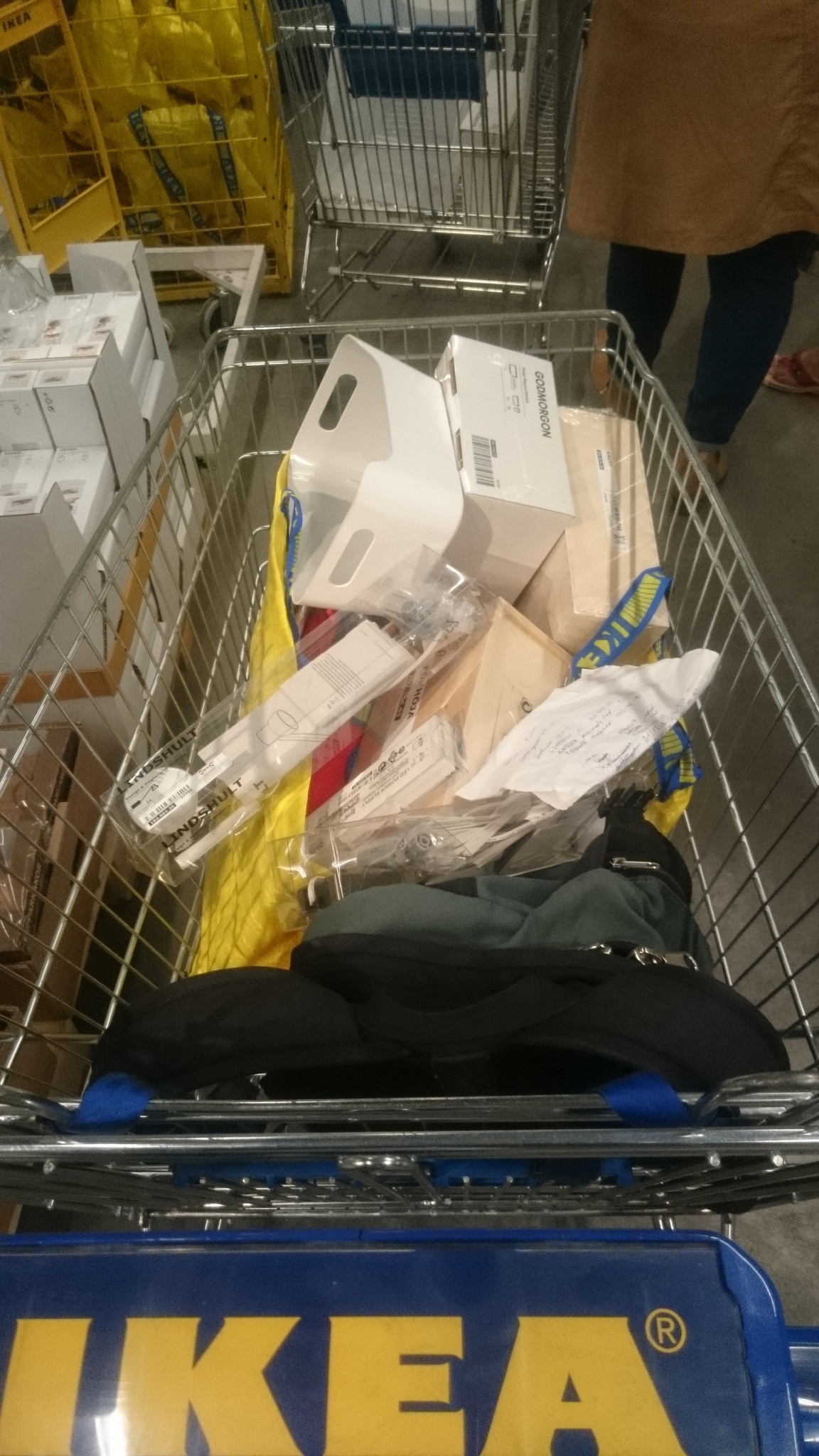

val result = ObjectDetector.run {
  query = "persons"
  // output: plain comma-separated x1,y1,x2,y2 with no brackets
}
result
763,348,819,395
565,0,819,518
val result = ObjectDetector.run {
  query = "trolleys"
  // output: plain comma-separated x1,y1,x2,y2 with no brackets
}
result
0,308,819,1456
0,0,300,343
248,0,595,360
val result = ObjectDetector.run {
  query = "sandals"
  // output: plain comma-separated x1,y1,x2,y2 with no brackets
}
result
762,349,819,393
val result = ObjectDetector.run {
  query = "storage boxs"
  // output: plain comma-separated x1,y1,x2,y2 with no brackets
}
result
0,241,673,1235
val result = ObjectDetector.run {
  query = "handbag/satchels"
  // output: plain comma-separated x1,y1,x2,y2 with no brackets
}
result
302,817,714,977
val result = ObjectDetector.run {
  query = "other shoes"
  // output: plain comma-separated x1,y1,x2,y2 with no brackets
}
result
669,440,729,516
591,328,638,422
